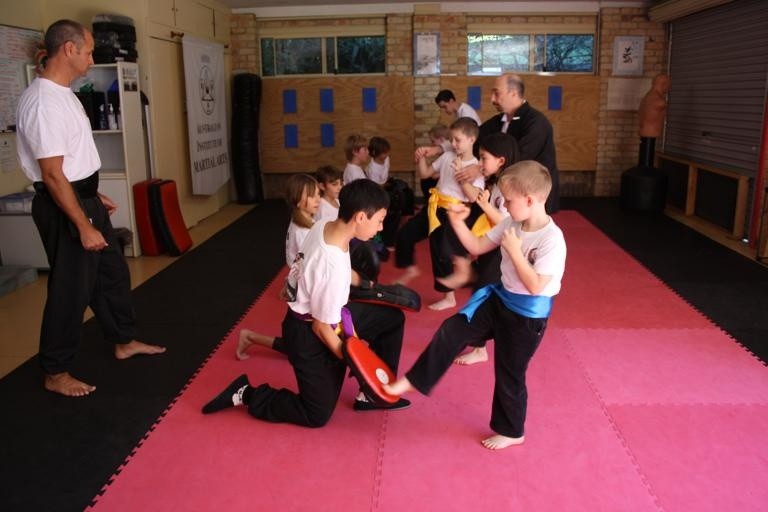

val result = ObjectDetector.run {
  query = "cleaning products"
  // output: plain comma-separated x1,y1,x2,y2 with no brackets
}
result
117,104,122,130
106,104,118,130
97,103,108,130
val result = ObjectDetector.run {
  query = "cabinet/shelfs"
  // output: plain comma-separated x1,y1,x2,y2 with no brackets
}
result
26,62,149,259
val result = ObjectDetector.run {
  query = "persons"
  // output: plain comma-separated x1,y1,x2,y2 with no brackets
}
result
389,73,559,368
383,162,569,452
236,133,415,360
14,19,166,398
202,180,411,430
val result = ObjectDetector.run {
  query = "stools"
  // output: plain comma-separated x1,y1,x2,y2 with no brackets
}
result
0,213,52,271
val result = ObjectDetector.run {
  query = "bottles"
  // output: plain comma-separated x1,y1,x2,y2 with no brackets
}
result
98,104,122,130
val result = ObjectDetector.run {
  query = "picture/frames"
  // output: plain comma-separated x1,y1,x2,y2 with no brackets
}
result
413,32,440,77
611,35,646,77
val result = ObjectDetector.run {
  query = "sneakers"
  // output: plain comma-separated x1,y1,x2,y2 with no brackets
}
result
353,397,411,411
202,375,250,413
